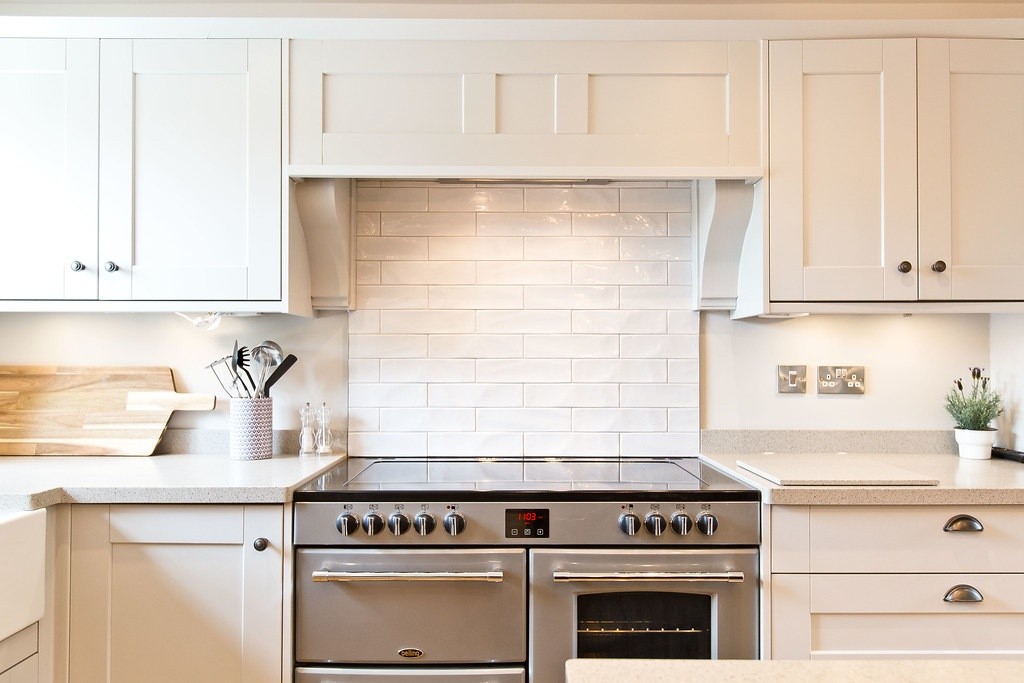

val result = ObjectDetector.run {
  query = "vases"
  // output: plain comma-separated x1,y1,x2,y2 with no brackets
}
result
955,427,999,459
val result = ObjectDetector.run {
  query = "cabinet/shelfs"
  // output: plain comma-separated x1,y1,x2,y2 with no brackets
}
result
0,36,283,301
772,504,1024,658
769,36,1024,303
69,503,282,683
0,622,39,682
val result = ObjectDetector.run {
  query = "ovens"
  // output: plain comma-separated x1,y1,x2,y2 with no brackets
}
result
294,550,763,682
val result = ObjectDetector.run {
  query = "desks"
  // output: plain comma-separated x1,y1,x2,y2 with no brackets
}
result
565,657,1024,682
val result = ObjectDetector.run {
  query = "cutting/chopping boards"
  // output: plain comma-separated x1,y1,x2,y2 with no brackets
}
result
0,364,216,455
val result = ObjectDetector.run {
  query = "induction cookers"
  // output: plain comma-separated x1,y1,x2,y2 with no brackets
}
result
294,455,761,546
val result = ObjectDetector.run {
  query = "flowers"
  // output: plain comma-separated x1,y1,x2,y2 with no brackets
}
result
943,366,1005,430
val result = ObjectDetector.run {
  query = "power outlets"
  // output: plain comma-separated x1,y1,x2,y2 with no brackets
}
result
817,367,865,394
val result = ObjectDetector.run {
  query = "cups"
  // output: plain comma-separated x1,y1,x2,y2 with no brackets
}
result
236,401,272,460
